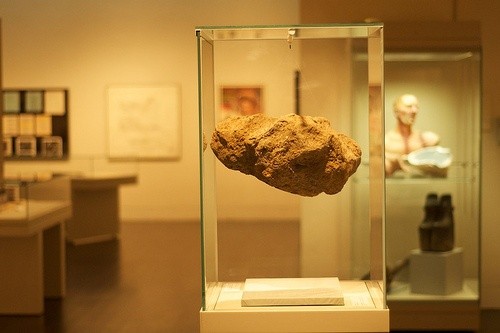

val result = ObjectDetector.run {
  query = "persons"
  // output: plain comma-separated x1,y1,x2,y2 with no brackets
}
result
384,92,440,176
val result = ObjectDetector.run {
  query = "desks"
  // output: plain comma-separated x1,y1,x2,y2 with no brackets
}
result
0,174,71,318
69,174,140,249
